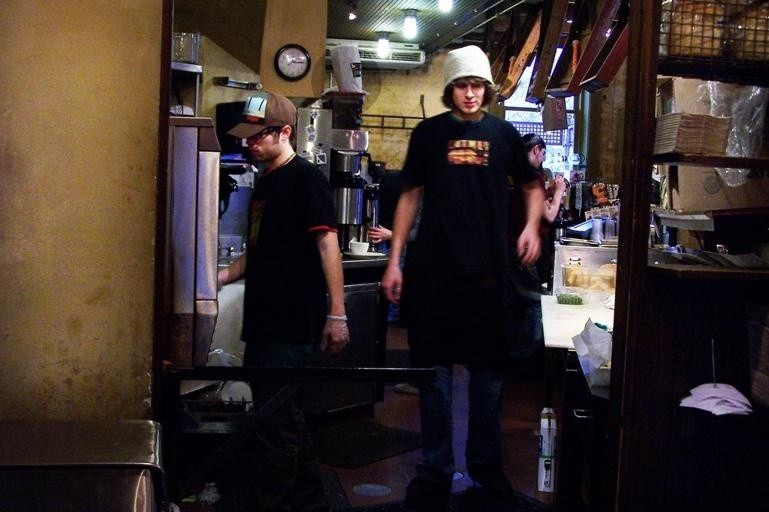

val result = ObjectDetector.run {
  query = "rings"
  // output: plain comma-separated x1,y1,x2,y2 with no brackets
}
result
396,286,402,289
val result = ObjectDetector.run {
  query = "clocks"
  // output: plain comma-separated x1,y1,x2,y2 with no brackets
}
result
274,44,312,82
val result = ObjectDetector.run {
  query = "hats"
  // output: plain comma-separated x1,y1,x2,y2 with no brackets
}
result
443,46,495,95
227,92,297,139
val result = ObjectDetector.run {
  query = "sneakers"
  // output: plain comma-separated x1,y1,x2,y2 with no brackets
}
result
406,471,454,495
467,465,512,489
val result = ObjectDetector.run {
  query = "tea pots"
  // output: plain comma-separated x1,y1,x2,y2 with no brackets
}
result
568,153,585,164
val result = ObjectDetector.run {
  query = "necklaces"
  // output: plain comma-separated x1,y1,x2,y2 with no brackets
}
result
261,149,297,176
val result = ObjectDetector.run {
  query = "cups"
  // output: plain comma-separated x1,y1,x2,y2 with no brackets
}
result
591,218,615,244
349,242,369,254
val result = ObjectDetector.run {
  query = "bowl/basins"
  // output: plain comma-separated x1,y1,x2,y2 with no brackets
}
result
556,288,584,305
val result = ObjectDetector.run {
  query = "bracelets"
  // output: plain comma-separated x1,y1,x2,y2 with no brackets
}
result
326,312,349,324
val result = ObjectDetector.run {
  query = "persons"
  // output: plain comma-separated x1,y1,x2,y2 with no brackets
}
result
217,88,353,512
500,131,568,385
365,184,422,396
379,76,549,512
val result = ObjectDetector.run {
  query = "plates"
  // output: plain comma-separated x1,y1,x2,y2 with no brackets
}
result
344,251,385,260
653,109,732,156
